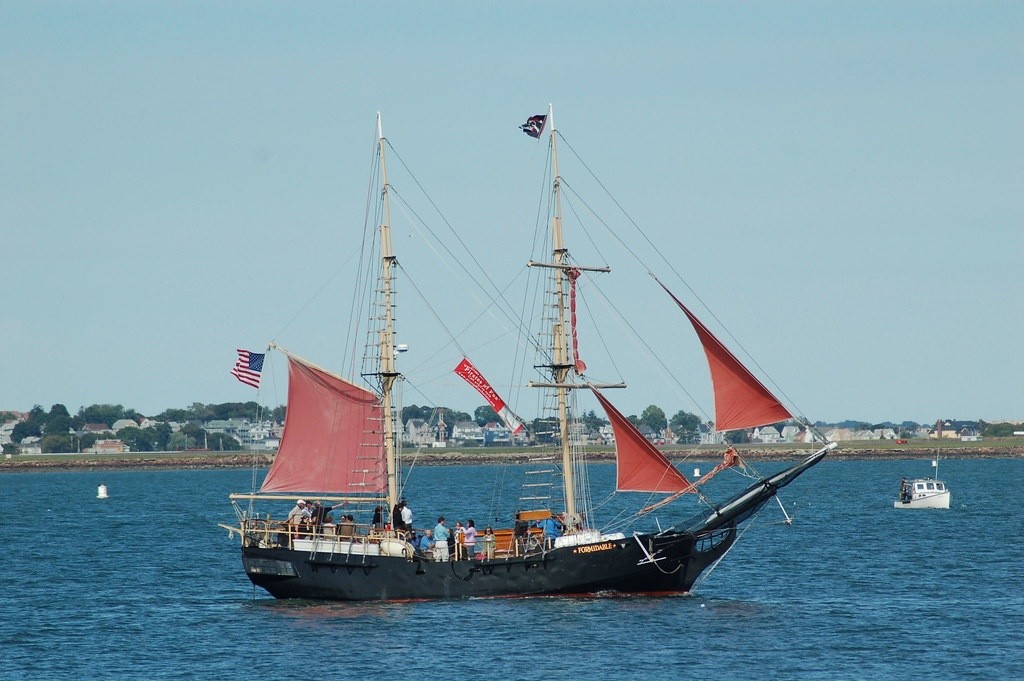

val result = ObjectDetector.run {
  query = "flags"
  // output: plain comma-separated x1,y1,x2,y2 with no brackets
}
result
230,348,266,389
518,114,548,139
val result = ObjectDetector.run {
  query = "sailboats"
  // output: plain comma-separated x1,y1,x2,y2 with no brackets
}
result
218,103,840,605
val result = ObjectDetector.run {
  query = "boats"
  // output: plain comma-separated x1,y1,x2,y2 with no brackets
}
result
891,418,950,510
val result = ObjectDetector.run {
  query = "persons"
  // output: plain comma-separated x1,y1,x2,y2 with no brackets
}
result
288,500,363,544
514,513,564,544
481,527,496,559
372,499,478,562
900,477,907,491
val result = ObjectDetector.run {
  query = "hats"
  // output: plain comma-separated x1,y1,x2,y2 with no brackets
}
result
297,499,306,505
